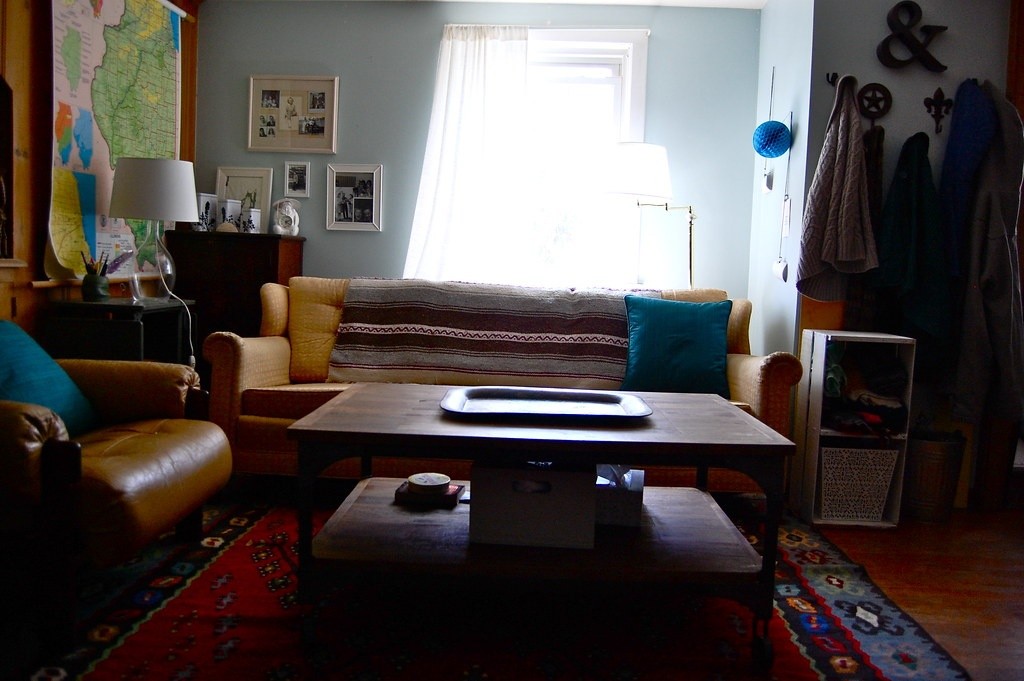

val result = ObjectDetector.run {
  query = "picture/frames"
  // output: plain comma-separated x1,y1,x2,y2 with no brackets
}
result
325,161,384,234
211,166,274,232
242,73,341,154
283,158,311,198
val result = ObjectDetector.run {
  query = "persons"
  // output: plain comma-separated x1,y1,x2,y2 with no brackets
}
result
311,94,323,109
262,94,279,108
285,97,297,128
292,171,298,190
336,192,354,221
259,115,275,137
302,116,316,134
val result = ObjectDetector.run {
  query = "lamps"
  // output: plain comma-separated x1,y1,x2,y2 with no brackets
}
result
102,155,202,301
606,136,700,290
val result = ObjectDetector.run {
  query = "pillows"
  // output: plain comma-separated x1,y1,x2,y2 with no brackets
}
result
0,320,97,438
619,293,738,398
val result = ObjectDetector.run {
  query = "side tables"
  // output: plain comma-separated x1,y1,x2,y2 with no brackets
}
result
47,296,200,365
797,326,921,529
163,231,308,356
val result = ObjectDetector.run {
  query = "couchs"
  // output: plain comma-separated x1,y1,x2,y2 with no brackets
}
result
197,276,804,519
0,317,236,602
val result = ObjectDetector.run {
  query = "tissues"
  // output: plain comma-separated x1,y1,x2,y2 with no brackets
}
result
596,463,645,524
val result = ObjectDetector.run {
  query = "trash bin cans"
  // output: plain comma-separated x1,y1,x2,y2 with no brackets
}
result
900,431,967,522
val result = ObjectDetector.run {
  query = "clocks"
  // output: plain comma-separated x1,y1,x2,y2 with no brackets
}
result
271,197,306,235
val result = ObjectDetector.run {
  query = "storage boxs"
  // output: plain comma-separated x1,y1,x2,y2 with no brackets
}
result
470,456,596,551
195,190,218,229
595,464,647,528
216,198,242,228
239,207,261,233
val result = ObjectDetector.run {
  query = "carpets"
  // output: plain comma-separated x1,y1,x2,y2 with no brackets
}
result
25,485,985,681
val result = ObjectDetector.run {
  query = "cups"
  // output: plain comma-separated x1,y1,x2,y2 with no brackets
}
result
81,275,109,302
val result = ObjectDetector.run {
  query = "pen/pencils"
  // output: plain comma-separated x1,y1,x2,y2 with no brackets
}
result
86,252,109,276
81,251,87,265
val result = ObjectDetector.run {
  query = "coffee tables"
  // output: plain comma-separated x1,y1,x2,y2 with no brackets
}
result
279,377,805,674
